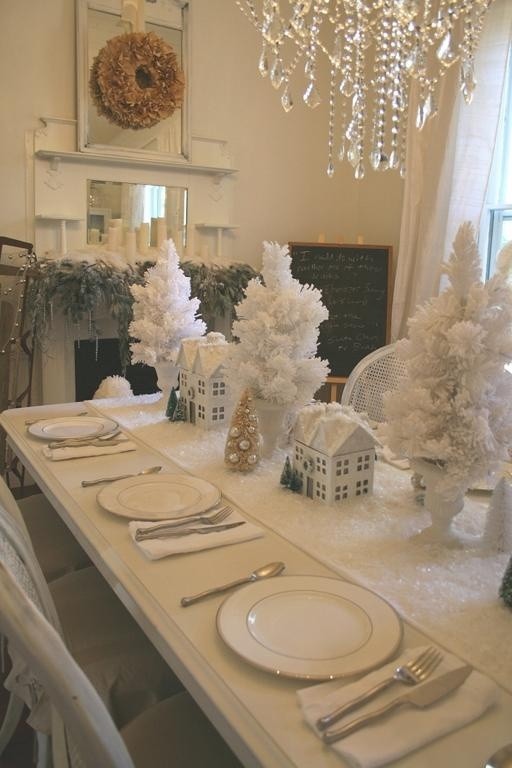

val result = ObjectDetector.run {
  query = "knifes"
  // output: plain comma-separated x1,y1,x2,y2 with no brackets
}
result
48,438,131,447
134,521,246,543
319,663,474,745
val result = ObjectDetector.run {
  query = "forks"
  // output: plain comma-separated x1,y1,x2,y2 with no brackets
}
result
136,505,234,535
56,431,122,441
315,644,443,732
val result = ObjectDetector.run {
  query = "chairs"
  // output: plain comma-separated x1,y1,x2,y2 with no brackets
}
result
0,478,238,768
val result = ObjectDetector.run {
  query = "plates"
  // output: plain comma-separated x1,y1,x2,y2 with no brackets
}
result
467,459,512,492
95,473,222,522
215,574,405,683
28,416,119,441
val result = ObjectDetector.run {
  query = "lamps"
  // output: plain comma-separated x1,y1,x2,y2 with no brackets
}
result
231,1,494,181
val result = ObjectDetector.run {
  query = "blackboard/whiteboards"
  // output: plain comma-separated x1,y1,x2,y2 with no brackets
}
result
289,242,393,384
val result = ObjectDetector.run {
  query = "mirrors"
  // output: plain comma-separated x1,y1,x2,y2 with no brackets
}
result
83,180,190,254
81,2,192,159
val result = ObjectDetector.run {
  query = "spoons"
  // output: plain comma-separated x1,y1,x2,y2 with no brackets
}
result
25,411,89,425
81,465,162,488
181,560,285,608
